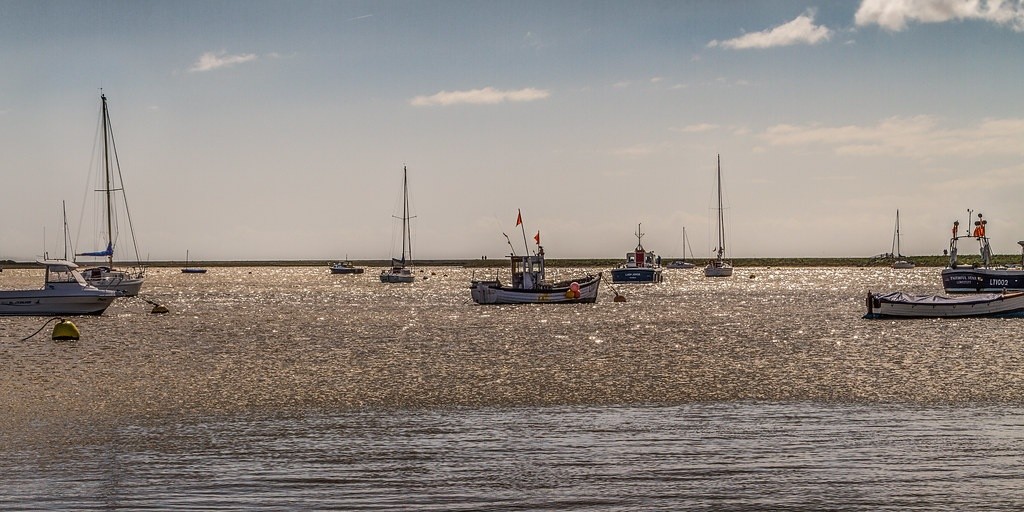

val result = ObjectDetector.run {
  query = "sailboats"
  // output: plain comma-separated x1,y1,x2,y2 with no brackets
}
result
380,166,416,283
46,87,144,297
704,153,734,277
890,208,915,268
666,226,696,268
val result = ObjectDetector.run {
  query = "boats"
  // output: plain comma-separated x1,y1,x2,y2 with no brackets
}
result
180,249,207,274
0,258,117,316
330,257,364,274
861,286,1024,319
468,208,603,305
941,208,1024,294
611,223,663,284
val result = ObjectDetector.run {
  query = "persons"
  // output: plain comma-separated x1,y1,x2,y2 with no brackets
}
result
657,255,661,268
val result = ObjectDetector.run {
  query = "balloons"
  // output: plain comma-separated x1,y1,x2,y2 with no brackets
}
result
566,282,580,298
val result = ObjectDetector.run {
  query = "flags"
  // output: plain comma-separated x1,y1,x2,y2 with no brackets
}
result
533,232,539,244
515,211,521,227
